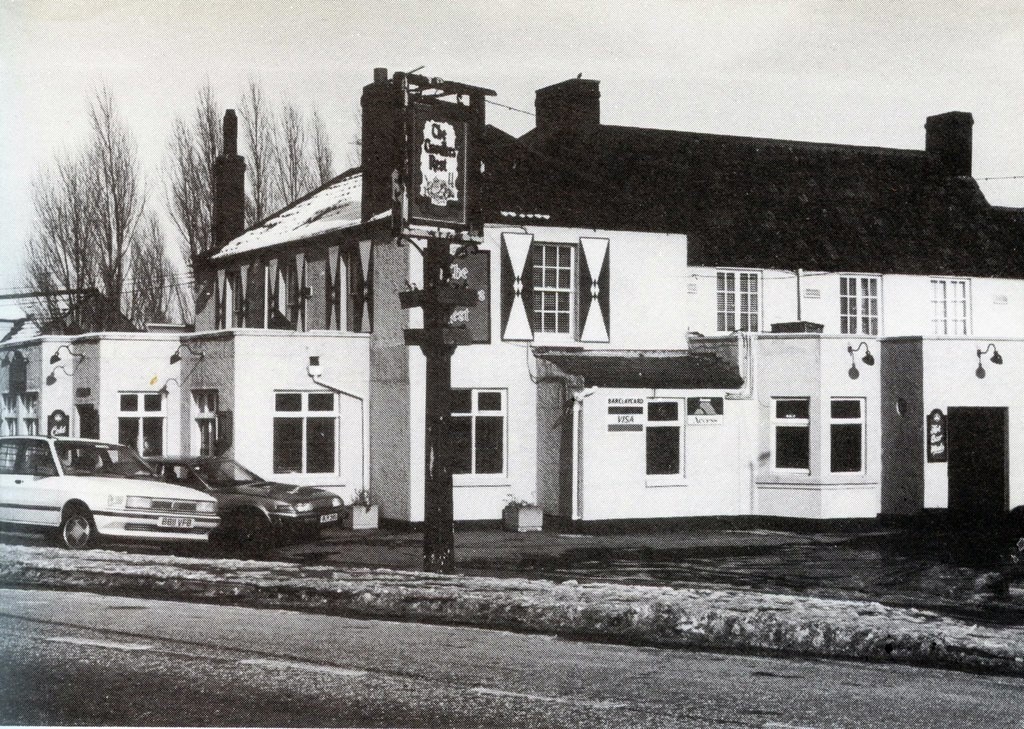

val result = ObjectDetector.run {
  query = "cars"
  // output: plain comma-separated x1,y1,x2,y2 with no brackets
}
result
0,435,220,551
102,456,347,561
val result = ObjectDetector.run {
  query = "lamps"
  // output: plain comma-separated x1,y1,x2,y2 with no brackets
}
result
849,341,874,366
49,345,86,365
170,344,204,364
977,343,1003,364
